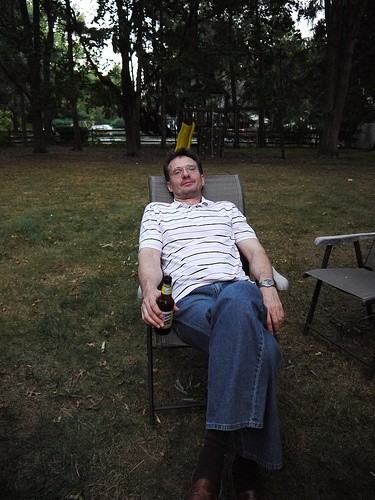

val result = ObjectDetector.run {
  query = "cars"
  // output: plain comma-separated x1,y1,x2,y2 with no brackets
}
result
227,126,259,140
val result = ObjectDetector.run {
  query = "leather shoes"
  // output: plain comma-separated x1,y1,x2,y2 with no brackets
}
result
227,460,266,500
187,467,222,500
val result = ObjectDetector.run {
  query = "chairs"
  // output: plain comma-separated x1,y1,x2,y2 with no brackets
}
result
138,174,288,427
303,233,375,379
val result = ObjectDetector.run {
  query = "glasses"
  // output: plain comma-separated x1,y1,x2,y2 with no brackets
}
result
169,165,200,178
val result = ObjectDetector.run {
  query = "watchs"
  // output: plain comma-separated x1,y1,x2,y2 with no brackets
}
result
259,279,276,288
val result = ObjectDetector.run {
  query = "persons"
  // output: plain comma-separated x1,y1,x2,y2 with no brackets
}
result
138,147,285,500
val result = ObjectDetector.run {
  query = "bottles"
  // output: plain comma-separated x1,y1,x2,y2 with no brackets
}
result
153,275,174,336
